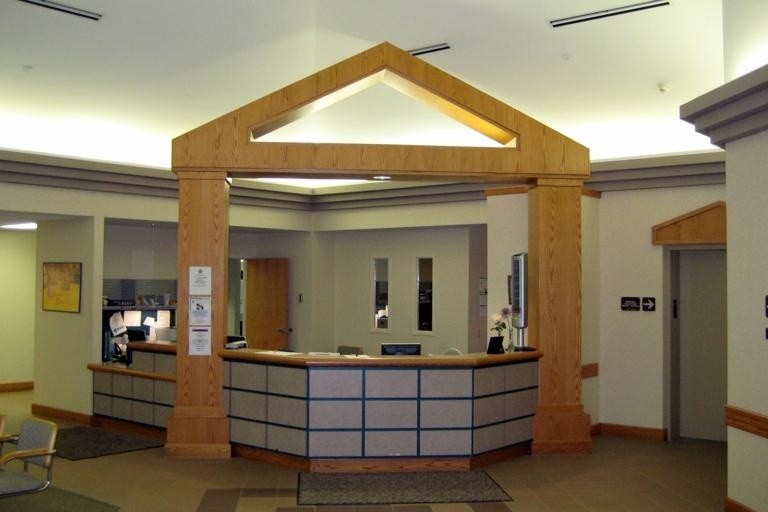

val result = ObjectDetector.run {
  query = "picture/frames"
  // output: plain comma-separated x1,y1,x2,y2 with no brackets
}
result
41,260,82,314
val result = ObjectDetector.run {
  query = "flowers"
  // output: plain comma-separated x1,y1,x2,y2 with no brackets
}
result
500,304,521,351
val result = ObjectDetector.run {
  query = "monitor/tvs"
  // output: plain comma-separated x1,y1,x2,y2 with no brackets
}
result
125,326,147,341
381,343,422,355
511,252,535,351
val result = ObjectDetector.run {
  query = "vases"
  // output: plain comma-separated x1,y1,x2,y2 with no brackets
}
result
506,327,515,355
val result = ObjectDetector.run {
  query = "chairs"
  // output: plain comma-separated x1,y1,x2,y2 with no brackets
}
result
336,345,362,357
0,414,58,497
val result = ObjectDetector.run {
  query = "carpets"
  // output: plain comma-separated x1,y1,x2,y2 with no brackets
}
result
9,424,165,462
295,471,513,507
0,480,124,512
